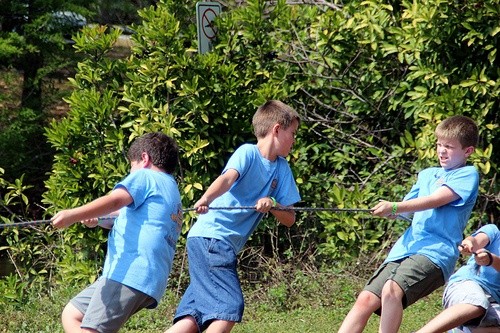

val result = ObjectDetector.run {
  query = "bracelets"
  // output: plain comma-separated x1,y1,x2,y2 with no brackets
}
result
486,252,492,267
272,197,277,208
392,202,397,216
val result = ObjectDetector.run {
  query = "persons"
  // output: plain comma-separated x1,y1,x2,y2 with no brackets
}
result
416,224,500,333
336,115,480,333
50,131,183,333
166,100,307,333
